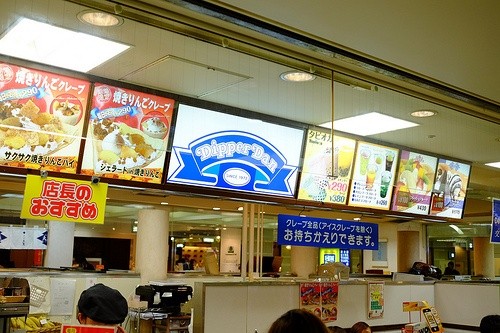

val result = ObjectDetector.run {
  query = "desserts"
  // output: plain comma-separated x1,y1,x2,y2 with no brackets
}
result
0,96,78,161
91,118,164,175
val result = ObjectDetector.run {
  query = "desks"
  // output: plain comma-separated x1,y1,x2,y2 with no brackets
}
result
0,272,140,320
192,278,500,333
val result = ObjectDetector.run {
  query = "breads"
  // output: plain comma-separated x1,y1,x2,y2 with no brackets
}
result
11,317,55,330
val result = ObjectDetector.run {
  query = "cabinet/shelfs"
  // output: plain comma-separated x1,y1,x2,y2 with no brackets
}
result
128,312,191,333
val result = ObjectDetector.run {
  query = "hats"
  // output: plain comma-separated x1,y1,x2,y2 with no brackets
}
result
78,283,128,324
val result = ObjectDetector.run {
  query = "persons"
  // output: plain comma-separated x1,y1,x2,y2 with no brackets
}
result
480,315,500,333
444,261,460,275
269,309,372,333
76,284,129,333
177,255,197,270
